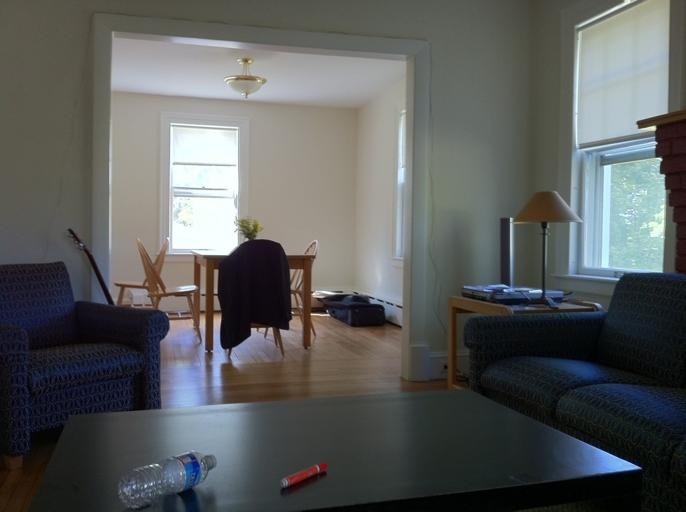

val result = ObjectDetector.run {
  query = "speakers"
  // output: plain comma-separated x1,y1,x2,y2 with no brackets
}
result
501,217,516,287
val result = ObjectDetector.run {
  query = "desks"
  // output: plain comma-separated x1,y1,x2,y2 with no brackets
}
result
192,249,316,352
446,294,601,389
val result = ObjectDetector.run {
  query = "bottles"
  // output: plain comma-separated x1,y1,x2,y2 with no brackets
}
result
117,450,217,510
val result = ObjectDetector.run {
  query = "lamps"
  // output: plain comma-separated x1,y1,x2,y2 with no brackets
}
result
509,189,583,311
223,59,269,99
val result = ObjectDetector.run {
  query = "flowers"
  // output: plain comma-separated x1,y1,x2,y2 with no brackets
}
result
231,215,262,241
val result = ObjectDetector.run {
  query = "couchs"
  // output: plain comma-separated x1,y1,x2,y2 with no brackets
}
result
460,271,684,512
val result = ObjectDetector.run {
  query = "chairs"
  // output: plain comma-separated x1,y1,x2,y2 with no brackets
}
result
114,237,171,305
215,239,292,357
136,237,203,343
253,238,319,339
0,257,170,455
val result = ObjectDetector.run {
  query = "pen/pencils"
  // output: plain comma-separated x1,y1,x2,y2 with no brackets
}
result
280,463,328,488
